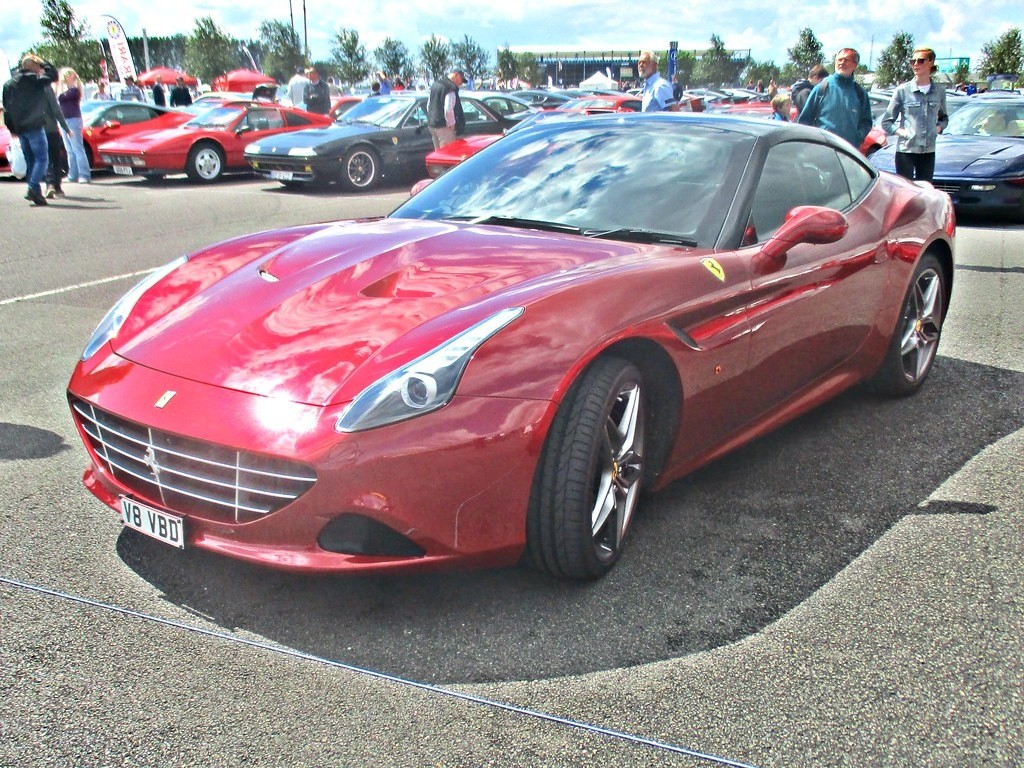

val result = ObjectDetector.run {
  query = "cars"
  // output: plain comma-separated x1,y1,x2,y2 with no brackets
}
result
190,92,251,109
243,97,522,192
330,90,1024,121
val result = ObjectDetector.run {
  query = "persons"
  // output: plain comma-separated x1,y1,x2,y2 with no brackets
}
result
56,68,91,184
1003,82,1010,90
92,74,193,107
880,48,949,184
962,79,987,96
2,55,74,205
328,70,426,98
670,74,683,106
746,63,830,124
464,82,543,91
303,67,331,115
426,68,466,151
287,65,311,113
637,51,681,112
796,47,872,150
615,79,644,93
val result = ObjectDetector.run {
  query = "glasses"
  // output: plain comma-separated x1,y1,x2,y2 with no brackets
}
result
909,58,929,66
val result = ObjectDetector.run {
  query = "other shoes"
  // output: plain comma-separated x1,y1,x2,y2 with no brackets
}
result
44,184,65,199
24,187,47,206
61,177,78,183
79,178,88,184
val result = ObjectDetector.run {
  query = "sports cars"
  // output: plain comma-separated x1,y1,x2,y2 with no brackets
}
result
63,100,957,584
0,100,197,174
868,94,1024,218
95,102,335,182
424,108,619,182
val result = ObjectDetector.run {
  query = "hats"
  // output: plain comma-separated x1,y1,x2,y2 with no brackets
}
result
176,76,183,82
124,75,134,80
450,68,469,84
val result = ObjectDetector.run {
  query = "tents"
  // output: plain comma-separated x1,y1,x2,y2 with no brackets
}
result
212,67,277,93
135,65,197,87
578,71,618,92
